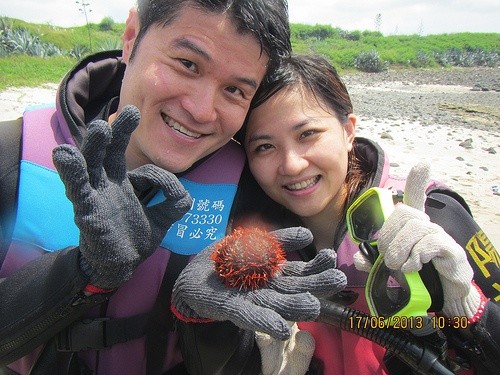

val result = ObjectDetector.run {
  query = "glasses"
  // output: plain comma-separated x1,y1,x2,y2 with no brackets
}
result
346,187,432,326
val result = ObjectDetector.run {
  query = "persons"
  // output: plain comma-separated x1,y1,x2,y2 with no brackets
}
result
0,0,294,375
177,55,499,374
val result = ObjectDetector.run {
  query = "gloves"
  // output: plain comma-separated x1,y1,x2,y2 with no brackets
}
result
51,104,192,292
255,311,315,375
352,163,478,324
171,226,348,340
